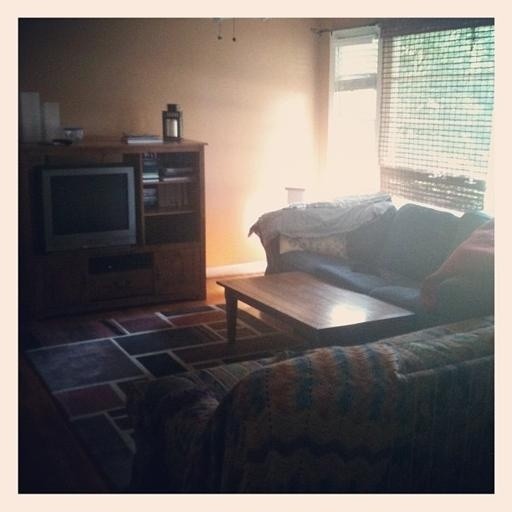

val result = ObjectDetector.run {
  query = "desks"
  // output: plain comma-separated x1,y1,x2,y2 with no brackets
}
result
217,269,417,358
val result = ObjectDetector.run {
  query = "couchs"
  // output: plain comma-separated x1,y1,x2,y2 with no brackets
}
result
160,315,495,492
246,196,494,323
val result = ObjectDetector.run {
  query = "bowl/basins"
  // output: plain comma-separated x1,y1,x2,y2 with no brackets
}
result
64,127,84,142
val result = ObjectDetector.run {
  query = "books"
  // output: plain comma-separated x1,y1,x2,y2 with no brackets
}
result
160,163,193,181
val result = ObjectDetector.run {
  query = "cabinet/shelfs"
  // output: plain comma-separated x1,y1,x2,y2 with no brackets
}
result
19,135,207,321
